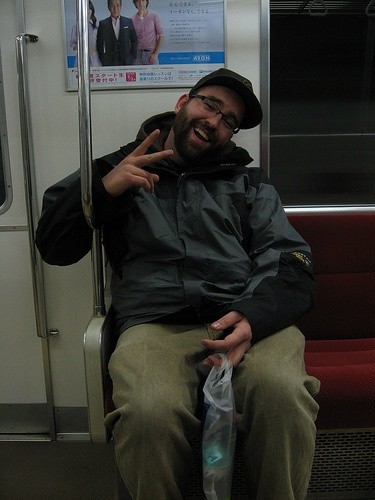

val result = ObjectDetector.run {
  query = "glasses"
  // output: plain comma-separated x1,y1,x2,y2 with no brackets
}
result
191,94,240,134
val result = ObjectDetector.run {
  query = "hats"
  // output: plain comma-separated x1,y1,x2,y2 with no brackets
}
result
190,68,263,130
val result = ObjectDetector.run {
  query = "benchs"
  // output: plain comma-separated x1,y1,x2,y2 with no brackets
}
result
81,206,375,500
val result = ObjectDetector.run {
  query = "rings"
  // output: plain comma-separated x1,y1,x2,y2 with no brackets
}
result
144,171,149,178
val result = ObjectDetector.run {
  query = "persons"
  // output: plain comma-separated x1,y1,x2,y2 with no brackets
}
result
34,68,321,500
69,0,165,68
